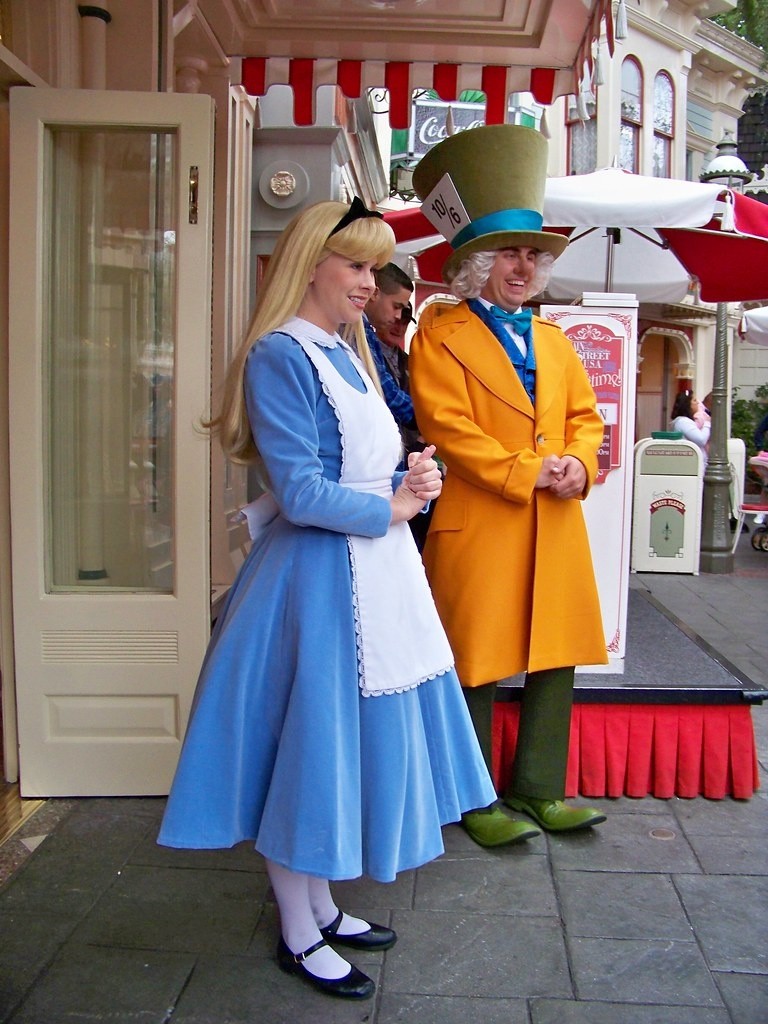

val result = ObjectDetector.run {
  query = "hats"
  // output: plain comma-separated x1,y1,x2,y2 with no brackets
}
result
411,125,570,286
402,301,417,324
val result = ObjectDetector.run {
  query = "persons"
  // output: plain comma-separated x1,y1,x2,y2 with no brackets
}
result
154,196,498,999
412,124,606,849
754,416,768,452
365,263,426,472
672,390,711,454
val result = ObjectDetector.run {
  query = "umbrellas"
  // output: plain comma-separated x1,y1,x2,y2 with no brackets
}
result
386,166,768,304
739,306,768,348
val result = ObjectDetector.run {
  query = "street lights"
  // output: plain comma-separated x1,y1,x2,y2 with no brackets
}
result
693,127,755,576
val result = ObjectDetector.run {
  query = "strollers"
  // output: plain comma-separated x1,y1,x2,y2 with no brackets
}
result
738,449,768,554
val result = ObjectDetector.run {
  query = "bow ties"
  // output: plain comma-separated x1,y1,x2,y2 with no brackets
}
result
490,305,535,336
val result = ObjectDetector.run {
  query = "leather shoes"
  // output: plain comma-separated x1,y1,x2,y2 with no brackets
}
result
462,793,611,848
276,908,397,1000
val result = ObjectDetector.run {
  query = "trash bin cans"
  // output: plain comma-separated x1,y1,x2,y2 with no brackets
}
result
630,432,703,576
